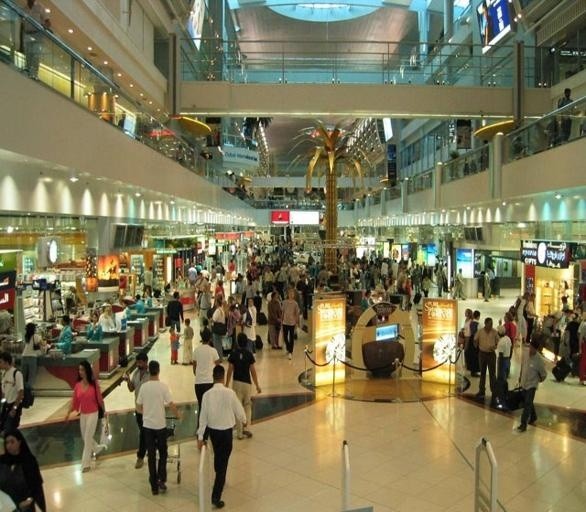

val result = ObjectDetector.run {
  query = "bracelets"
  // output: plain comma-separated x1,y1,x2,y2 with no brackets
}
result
27,497,34,502
13,405,18,410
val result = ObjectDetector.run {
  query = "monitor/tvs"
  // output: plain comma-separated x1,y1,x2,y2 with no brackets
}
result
186,0,206,50
476,0,517,55
376,325,399,341
382,118,394,142
290,211,320,225
272,211,289,224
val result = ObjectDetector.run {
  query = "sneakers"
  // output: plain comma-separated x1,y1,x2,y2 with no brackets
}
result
135,457,143,468
212,501,224,508
242,431,252,437
156,475,167,489
152,487,158,494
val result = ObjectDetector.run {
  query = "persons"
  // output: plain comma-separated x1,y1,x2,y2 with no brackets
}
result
135,359,182,494
118,111,277,210
19,0,50,81
477,0,493,48
62,360,110,473
516,338,545,434
19,322,48,388
189,333,259,438
0,431,45,512
0,352,25,433
196,366,246,509
50,234,585,406
121,352,158,469
462,87,586,177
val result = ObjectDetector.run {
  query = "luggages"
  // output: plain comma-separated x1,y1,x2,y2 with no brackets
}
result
533,318,543,351
256,335,263,350
552,353,576,382
256,311,267,325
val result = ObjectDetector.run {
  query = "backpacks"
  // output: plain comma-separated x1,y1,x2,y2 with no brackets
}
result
14,369,34,408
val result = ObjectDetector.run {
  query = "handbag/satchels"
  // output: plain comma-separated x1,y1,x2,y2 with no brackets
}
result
98,407,105,419
222,336,232,350
501,387,526,412
33,343,40,350
211,320,228,335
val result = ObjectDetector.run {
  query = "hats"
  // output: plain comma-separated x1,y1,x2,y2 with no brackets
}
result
530,340,540,350
497,325,506,335
